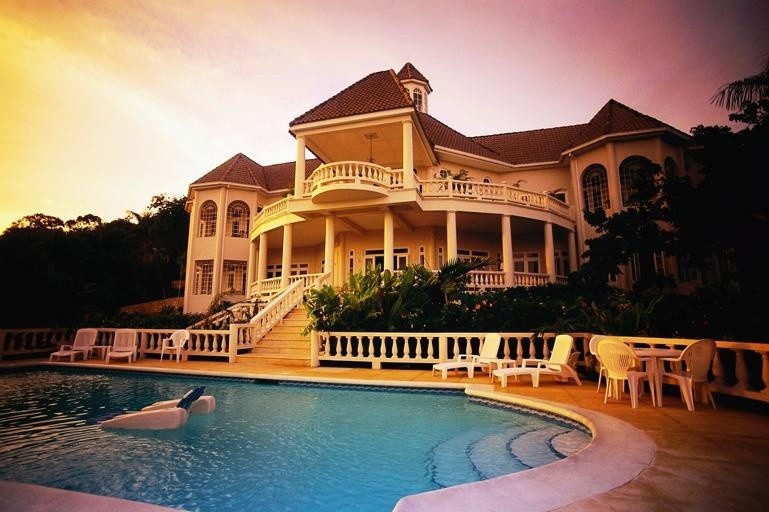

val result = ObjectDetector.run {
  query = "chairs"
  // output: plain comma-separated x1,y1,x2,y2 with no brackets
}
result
588,334,718,410
160,329,190,364
48,327,138,363
433,333,584,388
